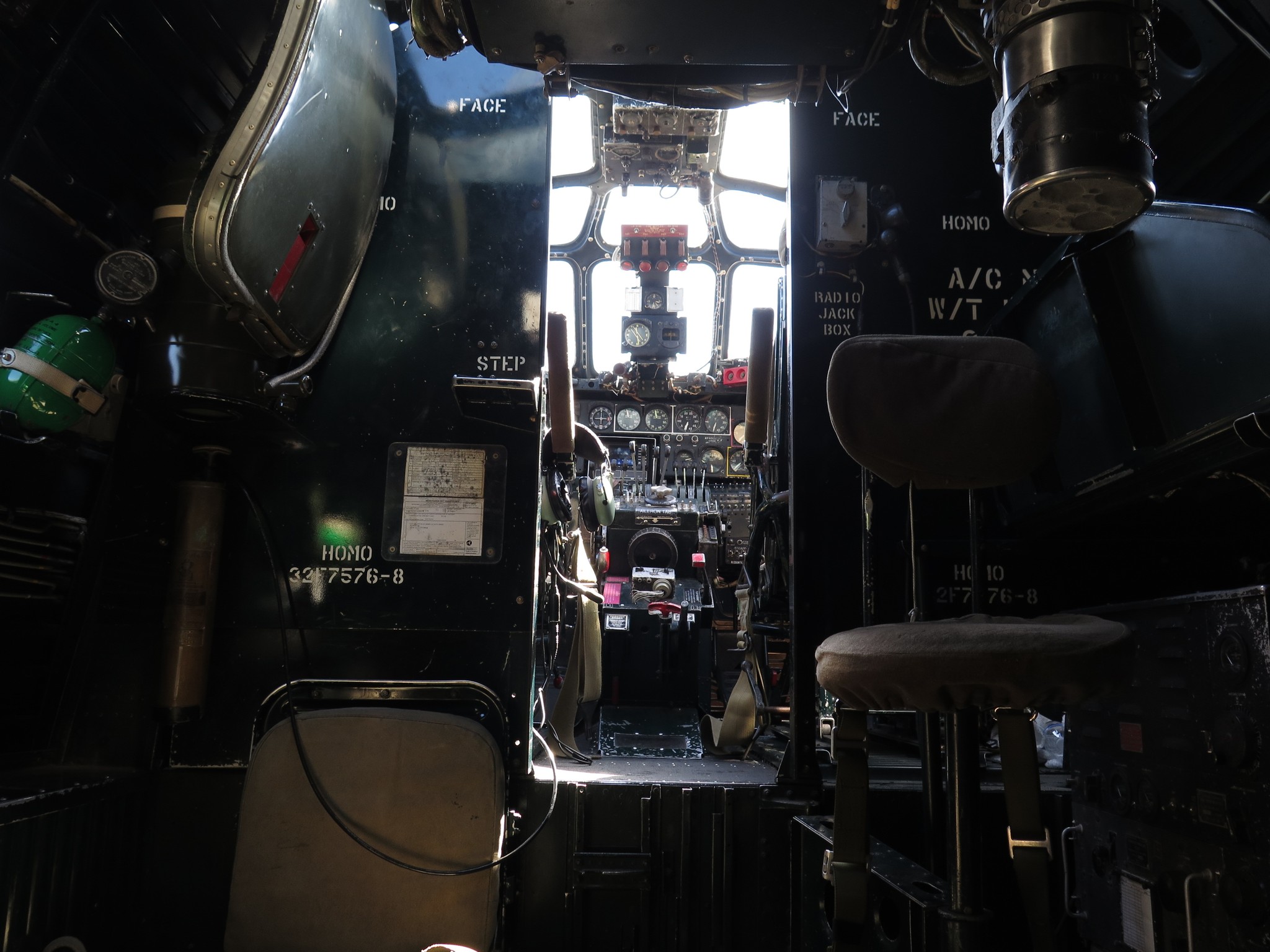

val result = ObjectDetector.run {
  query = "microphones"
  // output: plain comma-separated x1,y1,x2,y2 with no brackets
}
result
539,519,605,604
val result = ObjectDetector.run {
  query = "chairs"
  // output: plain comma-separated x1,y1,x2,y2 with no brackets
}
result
813,333,1129,952
225,677,513,952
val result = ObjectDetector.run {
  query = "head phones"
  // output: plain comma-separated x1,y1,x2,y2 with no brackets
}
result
541,421,616,533
